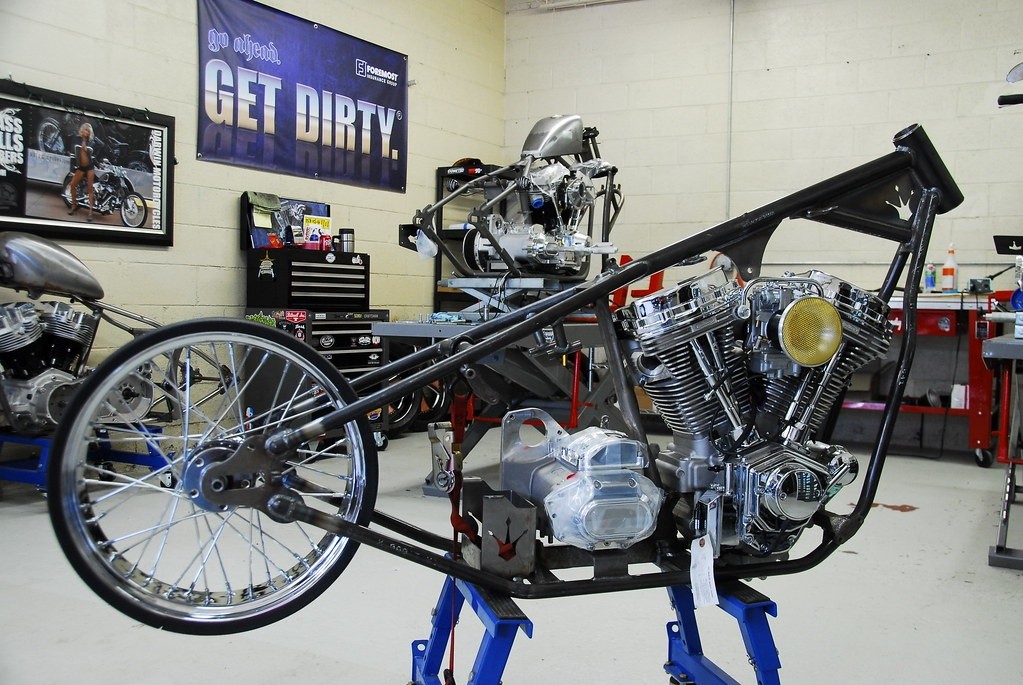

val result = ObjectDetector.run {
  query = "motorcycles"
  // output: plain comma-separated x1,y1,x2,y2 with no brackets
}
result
37,109,152,172
62,157,149,227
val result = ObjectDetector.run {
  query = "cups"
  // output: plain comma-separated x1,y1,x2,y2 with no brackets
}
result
332,228,354,252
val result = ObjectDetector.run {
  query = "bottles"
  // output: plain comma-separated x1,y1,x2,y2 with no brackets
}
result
941,250,959,294
924,265,937,295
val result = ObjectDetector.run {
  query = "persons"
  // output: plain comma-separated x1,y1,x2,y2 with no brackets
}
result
67,123,95,221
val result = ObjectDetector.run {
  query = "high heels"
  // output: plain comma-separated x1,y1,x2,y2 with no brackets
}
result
68,208,77,216
87,215,93,221
